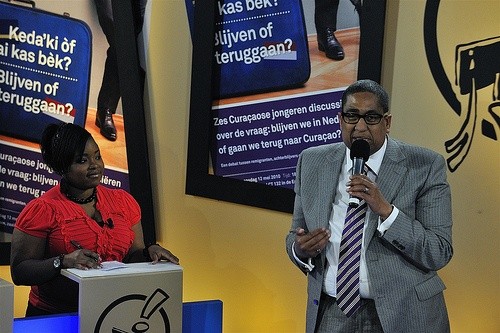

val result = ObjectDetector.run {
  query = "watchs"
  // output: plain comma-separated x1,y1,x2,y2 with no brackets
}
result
53,255,65,272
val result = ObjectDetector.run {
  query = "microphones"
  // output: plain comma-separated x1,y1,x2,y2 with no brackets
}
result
349,139,370,208
105,217,114,229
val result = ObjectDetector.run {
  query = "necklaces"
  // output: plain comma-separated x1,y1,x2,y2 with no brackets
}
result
60,177,97,204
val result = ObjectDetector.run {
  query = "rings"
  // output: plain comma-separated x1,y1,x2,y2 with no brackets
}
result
316,249,321,254
364,188,369,194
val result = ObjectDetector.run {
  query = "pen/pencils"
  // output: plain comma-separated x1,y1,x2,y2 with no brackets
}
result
70,240,103,268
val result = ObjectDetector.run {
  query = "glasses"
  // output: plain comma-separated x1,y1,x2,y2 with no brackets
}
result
340,110,384,126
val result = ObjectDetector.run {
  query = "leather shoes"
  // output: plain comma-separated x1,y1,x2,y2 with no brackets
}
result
317,26,345,61
94,104,117,142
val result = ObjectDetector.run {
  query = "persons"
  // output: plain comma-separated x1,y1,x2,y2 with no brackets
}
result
10,122,179,318
94,0,123,141
314,0,362,60
286,79,454,333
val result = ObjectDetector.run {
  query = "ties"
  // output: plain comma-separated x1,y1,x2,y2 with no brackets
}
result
336,165,369,319
